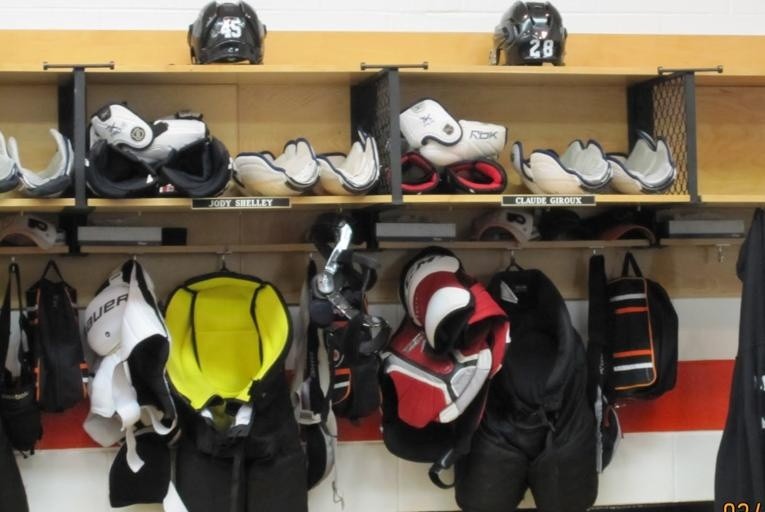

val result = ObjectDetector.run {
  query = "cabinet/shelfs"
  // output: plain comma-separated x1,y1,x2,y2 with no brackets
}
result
0,63,765,210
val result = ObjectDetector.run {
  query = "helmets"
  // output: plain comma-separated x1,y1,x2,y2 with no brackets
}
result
487,0,568,67
186,0,268,66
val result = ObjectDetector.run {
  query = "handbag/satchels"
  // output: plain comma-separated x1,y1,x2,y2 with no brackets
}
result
606,275,678,401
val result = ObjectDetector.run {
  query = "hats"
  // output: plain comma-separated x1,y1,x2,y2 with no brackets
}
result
465,206,657,246
